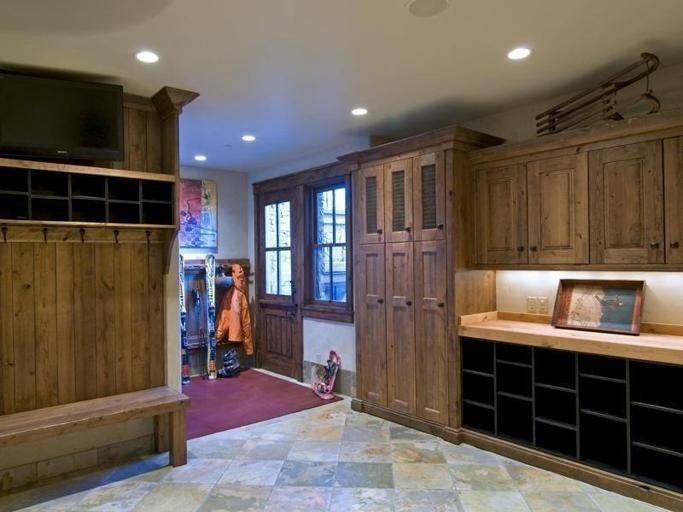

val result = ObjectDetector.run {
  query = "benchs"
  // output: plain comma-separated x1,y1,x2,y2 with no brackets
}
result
1,384,193,474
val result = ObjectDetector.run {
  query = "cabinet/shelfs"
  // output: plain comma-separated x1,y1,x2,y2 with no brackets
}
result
589,107,682,273
334,145,387,419
466,126,589,271
0,156,181,233
415,124,508,447
459,312,682,512
385,135,416,431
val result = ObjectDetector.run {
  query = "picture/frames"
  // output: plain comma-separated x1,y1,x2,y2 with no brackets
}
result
548,277,644,336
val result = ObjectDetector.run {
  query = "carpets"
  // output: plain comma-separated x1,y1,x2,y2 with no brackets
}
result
179,366,344,440
